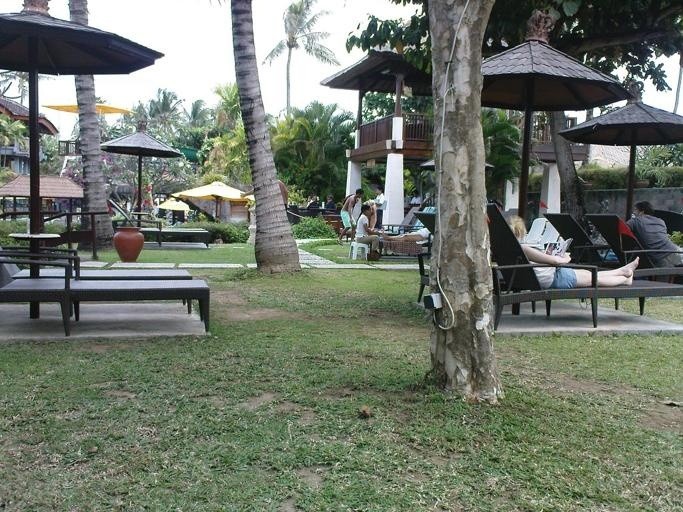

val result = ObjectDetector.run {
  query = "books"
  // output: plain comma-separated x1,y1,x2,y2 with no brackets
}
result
545,238,573,257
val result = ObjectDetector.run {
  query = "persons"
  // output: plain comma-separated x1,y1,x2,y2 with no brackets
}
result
506,216,640,289
298,185,434,260
240,179,289,214
625,201,683,267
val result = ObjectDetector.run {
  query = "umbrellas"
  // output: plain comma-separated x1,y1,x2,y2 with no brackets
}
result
479,40,635,220
42,105,137,113
558,103,683,222
156,197,194,225
172,181,255,223
0,11,165,319
100,129,183,227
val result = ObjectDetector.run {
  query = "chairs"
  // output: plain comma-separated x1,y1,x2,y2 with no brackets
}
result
0,218,210,336
416,203,682,328
415,204,683,329
